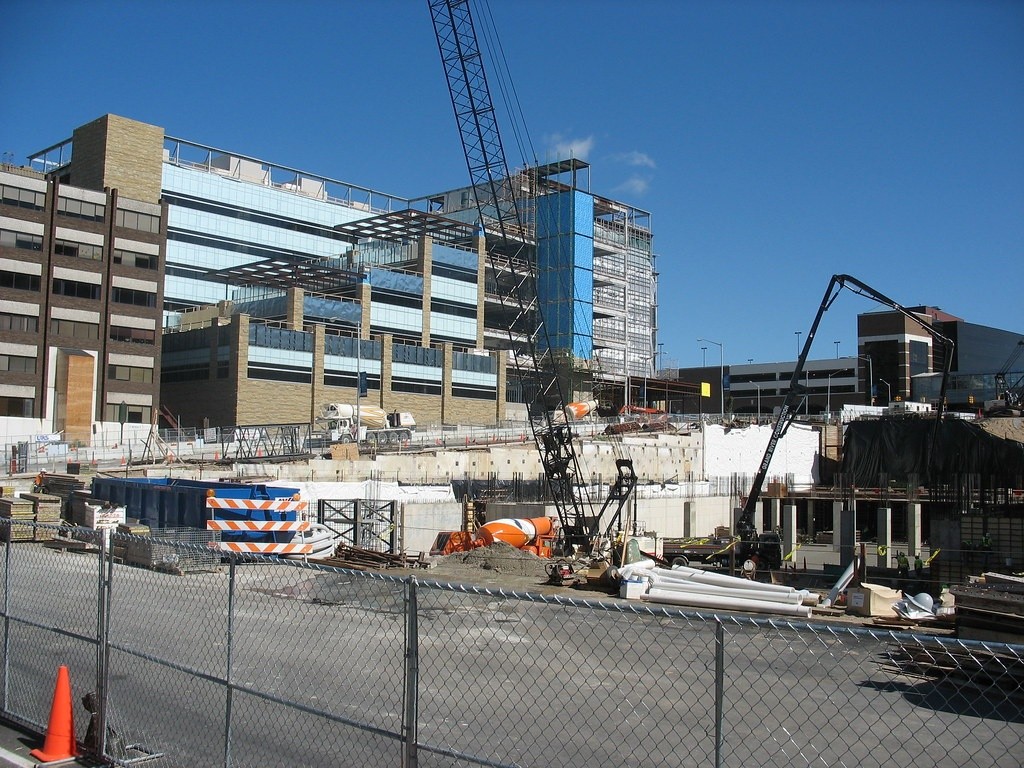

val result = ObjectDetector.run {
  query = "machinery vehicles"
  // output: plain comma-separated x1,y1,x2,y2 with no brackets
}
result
716,274,955,565
544,400,600,426
320,403,417,447
429,1,638,581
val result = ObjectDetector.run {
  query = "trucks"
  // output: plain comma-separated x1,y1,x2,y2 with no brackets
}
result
663,531,782,570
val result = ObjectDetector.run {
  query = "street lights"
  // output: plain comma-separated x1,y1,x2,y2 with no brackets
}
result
748,359,754,364
658,343,664,371
795,332,802,361
827,368,849,423
697,338,723,417
700,347,708,368
879,378,890,403
750,380,760,419
849,356,873,407
834,341,840,360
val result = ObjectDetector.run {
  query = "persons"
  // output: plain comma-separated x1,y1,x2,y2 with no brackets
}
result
913,555,923,580
750,551,762,565
897,551,910,579
33,467,48,487
960,532,994,550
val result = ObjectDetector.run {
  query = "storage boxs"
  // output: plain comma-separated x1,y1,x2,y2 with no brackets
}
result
848,588,902,616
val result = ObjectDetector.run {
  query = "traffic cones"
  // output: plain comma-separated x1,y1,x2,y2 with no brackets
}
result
31,665,84,762
214,450,218,460
121,456,127,465
257,448,263,457
